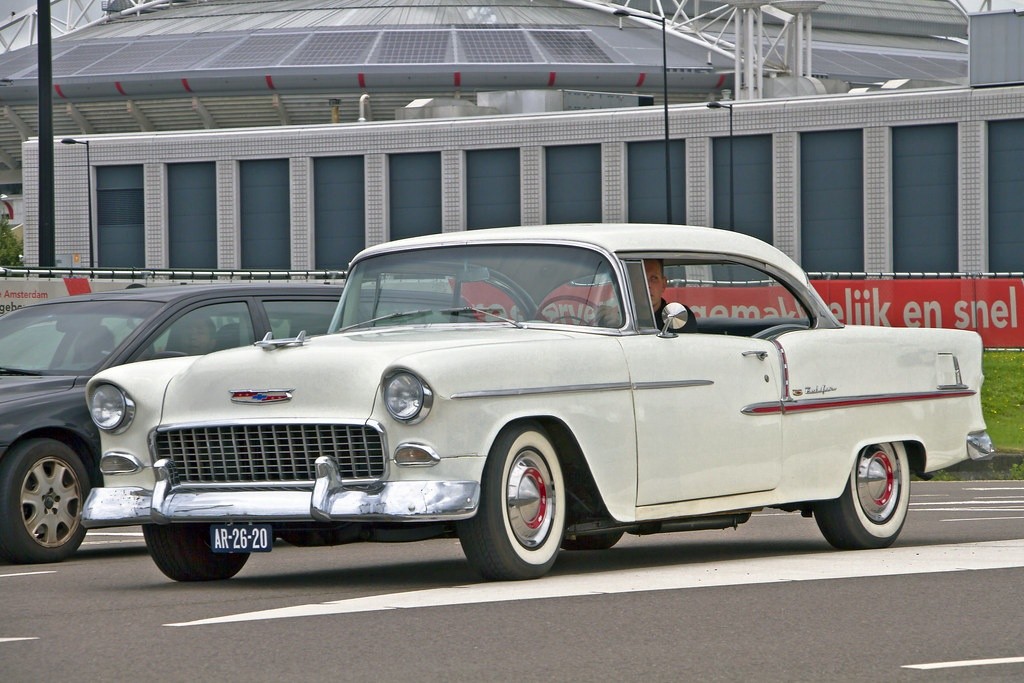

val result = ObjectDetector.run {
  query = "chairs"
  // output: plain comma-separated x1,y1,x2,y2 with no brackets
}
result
213,321,240,352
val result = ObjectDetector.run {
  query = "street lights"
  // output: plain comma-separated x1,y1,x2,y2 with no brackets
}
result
705,101,735,231
612,9,674,226
60,136,94,279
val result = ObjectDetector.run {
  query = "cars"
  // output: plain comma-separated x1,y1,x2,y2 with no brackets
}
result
0,278,473,565
78,221,997,584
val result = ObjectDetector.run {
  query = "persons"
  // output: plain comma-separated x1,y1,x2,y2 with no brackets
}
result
74,326,115,363
596,258,698,333
180,314,217,355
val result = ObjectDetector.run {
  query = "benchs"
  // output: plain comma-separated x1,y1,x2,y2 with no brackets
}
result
698,315,812,339
